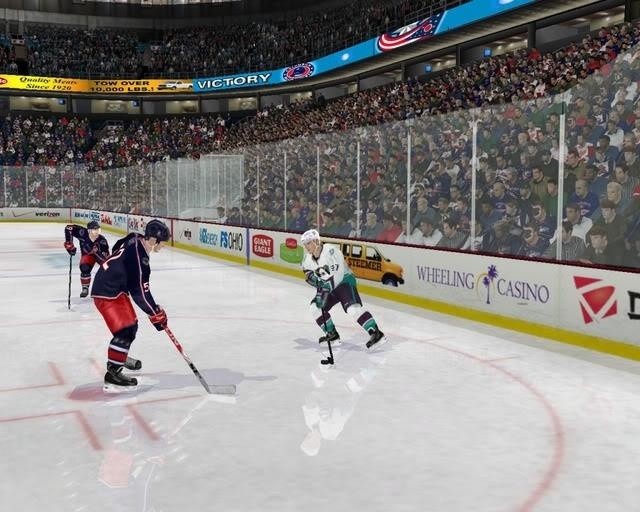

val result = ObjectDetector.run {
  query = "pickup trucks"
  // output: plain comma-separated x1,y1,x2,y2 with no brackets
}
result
157,81,193,90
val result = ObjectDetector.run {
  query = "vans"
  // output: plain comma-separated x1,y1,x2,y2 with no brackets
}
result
321,241,404,286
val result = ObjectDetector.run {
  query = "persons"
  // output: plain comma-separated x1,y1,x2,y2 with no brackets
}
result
1,1,639,269
299,227,386,351
63,220,112,299
89,217,172,390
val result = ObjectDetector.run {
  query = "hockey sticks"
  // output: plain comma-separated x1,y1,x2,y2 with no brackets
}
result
155,304,236,395
66,233,73,309
315,278,335,365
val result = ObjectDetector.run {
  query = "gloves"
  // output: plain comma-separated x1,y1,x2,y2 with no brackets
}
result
307,276,330,308
148,305,167,331
64,241,76,256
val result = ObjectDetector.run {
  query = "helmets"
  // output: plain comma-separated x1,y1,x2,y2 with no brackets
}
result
87,221,100,229
300,230,321,246
146,220,169,241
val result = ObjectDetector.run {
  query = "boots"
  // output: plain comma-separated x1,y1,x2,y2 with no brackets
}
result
366,325,384,348
105,361,138,386
125,356,141,370
80,285,89,297
320,325,339,342
